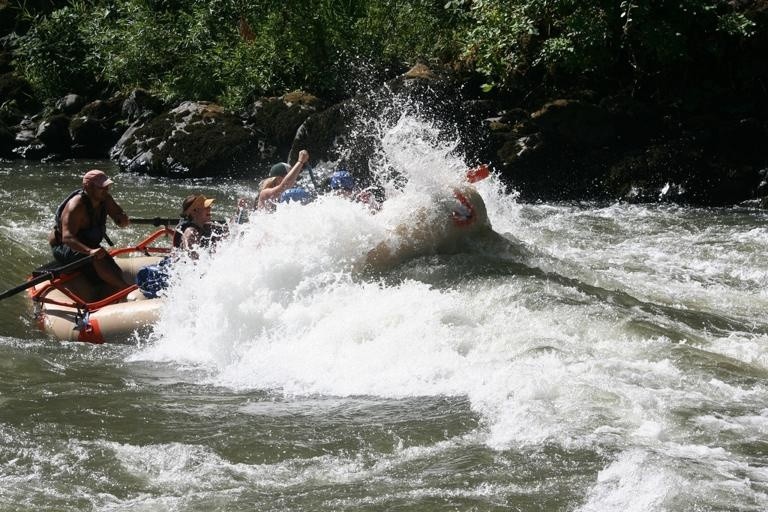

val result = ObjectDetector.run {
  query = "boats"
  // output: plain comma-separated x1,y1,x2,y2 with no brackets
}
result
24,182,492,343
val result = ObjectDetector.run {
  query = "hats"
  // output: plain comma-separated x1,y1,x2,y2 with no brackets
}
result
269,162,302,182
83,170,114,188
183,194,216,215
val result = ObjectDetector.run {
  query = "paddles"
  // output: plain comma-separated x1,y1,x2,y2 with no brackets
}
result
465,163,490,183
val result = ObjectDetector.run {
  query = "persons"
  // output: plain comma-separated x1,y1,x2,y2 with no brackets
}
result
173,192,232,261
49,170,134,291
254,149,490,215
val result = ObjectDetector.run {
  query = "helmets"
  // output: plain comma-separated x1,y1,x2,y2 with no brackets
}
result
280,189,306,202
330,171,354,189
